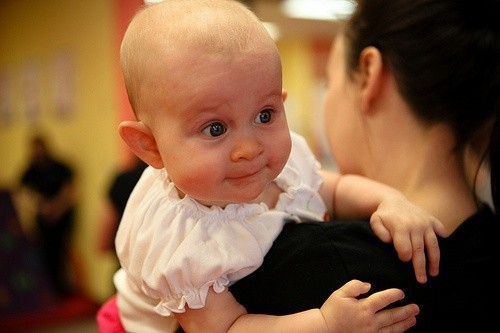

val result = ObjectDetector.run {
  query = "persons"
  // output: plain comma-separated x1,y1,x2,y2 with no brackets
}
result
174,0,500,333
0,135,147,320
94,0,447,333
